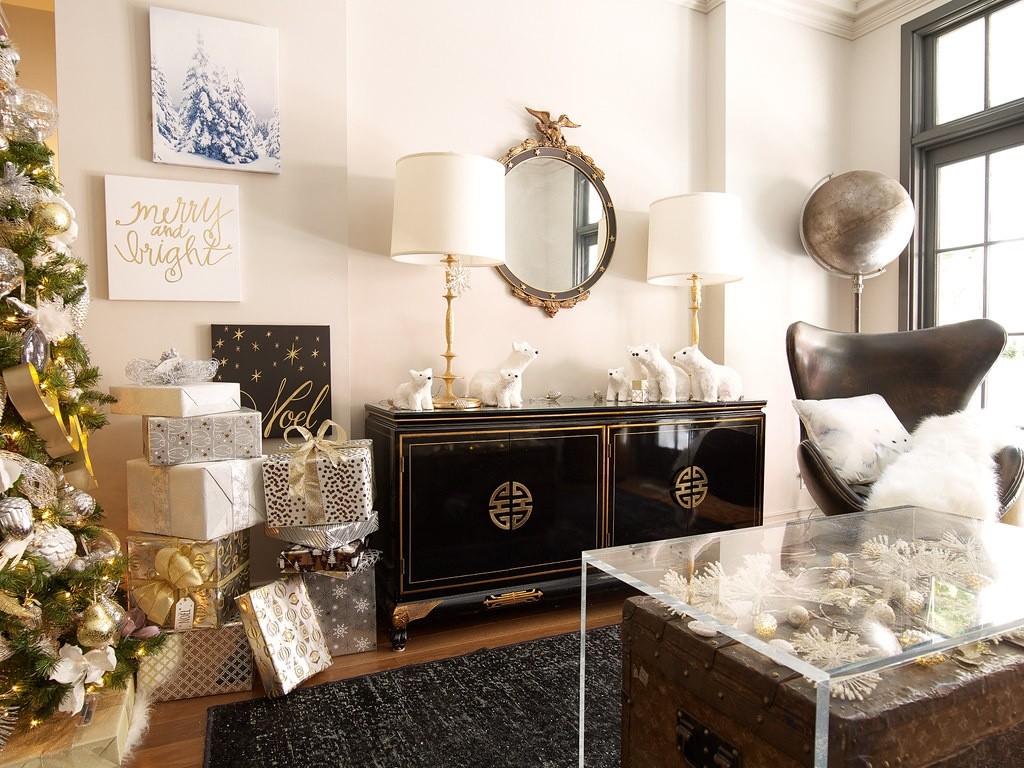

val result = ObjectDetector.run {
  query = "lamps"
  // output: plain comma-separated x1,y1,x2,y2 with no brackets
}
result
389,152,507,409
645,190,755,348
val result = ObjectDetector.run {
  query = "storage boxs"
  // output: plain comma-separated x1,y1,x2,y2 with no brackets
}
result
108,381,380,704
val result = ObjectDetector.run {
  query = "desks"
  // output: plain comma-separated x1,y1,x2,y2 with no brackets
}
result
576,505,1024,768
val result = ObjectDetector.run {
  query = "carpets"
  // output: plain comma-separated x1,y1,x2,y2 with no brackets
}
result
203,622,622,768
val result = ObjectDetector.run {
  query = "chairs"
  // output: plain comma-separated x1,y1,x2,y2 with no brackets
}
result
784,318,1024,517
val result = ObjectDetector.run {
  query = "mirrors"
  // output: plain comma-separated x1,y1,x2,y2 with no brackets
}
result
495,107,617,319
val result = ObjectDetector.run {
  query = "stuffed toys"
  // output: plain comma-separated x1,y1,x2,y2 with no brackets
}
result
864,407,1004,519
624,343,690,402
606,367,632,401
482,342,538,408
672,344,744,403
393,368,434,411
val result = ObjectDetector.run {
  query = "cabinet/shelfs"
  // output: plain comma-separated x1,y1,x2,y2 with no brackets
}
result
365,412,767,652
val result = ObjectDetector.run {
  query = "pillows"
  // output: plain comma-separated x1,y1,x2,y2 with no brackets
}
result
789,392,915,483
